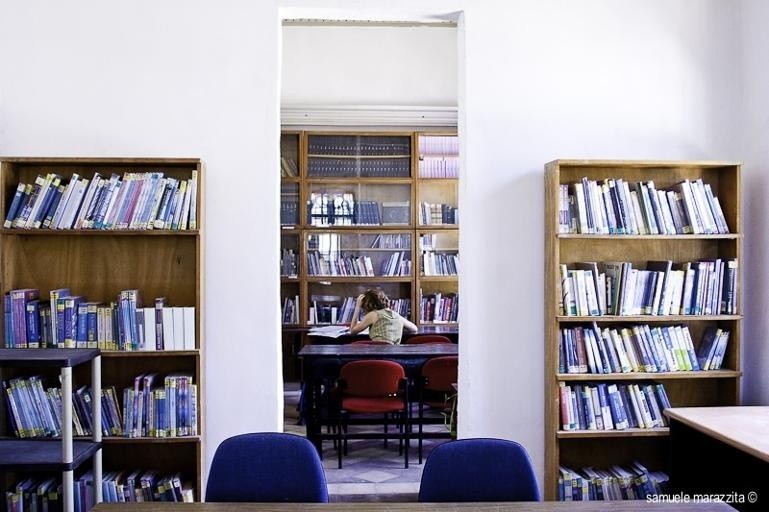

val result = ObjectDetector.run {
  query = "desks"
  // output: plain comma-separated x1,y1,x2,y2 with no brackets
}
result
663,403,769,512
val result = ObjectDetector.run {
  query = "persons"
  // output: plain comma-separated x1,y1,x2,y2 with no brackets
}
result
297,289,418,426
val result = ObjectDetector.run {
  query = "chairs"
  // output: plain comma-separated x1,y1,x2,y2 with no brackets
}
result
205,432,329,503
418,438,542,503
296,326,459,468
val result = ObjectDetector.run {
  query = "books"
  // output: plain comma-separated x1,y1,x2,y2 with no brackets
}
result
1,288,197,512
283,129,460,340
557,460,670,500
558,176,732,236
4,167,197,232
559,258,738,430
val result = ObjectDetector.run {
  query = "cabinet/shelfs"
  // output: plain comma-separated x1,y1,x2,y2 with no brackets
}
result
414,130,458,343
280,129,303,418
0,157,202,502
0,348,108,512
301,131,414,344
544,159,744,499
